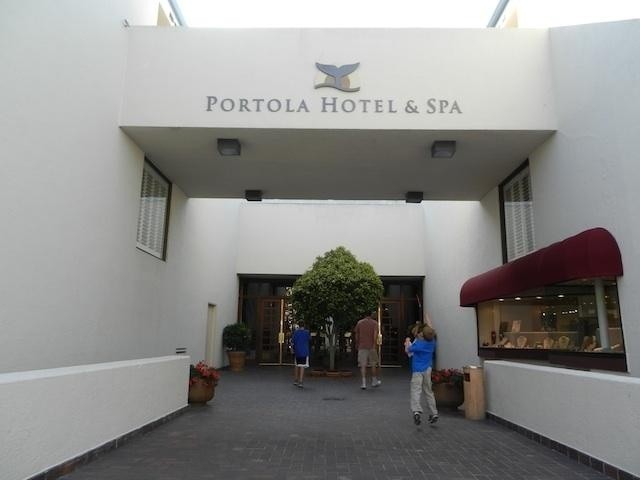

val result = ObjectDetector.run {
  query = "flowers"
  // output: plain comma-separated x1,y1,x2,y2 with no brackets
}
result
190,361,220,386
429,367,465,385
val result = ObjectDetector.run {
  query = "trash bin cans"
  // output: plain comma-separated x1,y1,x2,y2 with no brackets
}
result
463,366,486,420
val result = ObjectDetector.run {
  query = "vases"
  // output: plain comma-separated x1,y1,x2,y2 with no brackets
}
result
188,378,215,406
431,381,465,413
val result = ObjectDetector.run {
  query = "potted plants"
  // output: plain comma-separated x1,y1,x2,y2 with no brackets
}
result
221,323,254,371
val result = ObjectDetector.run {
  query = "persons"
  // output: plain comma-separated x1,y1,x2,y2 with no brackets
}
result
291,320,312,388
354,309,382,389
405,321,440,425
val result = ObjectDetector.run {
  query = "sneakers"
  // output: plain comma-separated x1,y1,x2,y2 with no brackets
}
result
292,379,305,389
412,413,439,427
360,380,382,390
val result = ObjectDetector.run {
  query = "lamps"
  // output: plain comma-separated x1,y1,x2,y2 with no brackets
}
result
430,140,456,158
216,139,241,157
244,190,263,202
405,191,423,204
